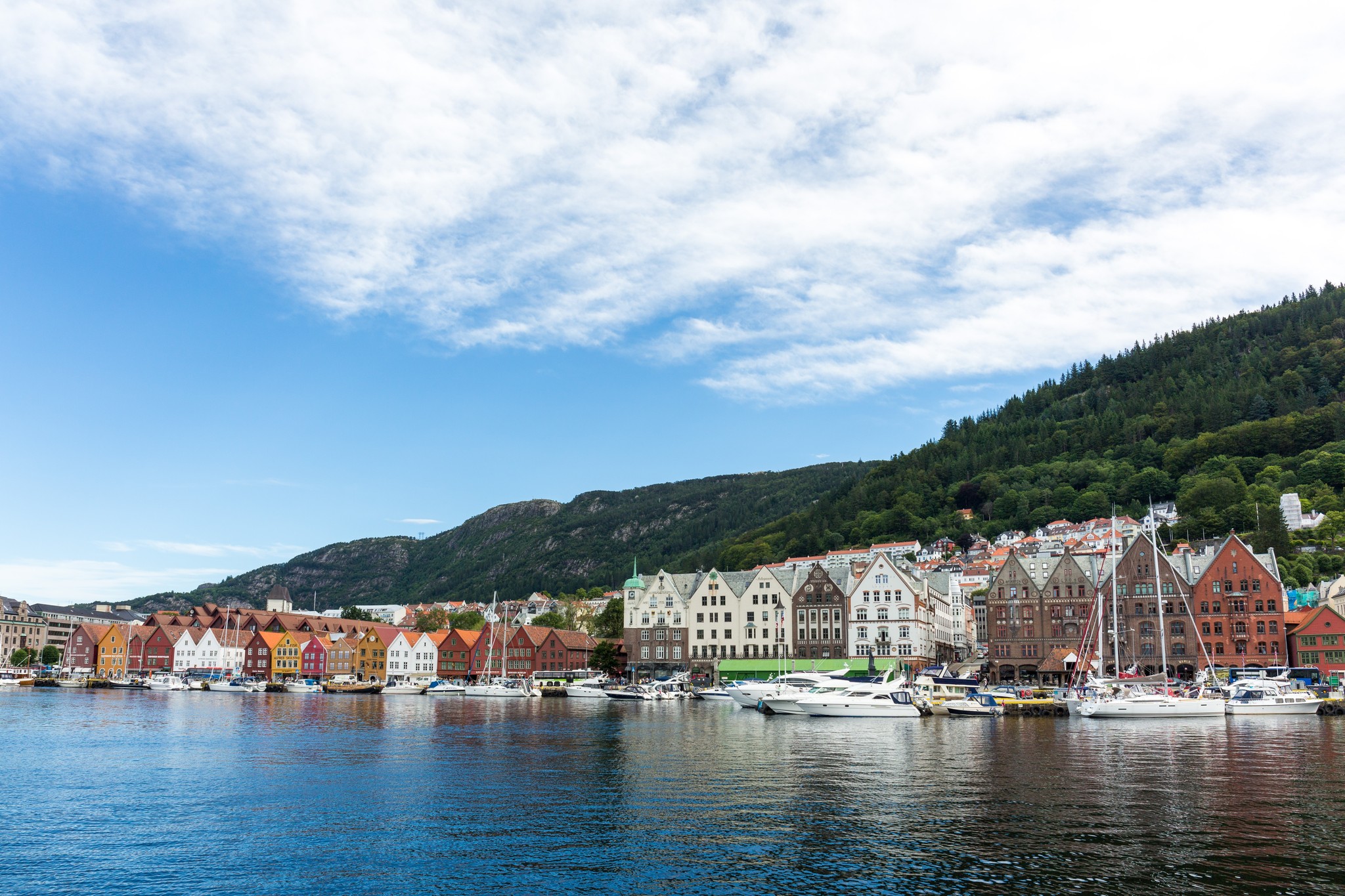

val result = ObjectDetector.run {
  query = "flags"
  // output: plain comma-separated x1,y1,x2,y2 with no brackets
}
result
975,669,977,673
974,673,977,680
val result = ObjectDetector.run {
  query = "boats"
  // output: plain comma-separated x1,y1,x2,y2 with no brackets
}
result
0,497,1345,721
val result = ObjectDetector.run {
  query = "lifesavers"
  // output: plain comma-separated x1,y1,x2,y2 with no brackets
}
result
35,680,58,687
204,685,209,691
1316,704,1345,715
1038,693,1046,699
135,680,138,683
14,674,18,678
542,690,568,697
109,682,113,687
92,682,107,688
266,685,283,692
1003,706,1066,717
764,705,774,715
1026,690,1032,696
328,682,330,685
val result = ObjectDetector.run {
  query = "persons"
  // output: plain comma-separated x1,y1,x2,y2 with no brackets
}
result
1014,676,1017,683
1,672,297,684
1091,688,1099,703
1064,683,1068,688
983,675,990,691
899,680,914,689
1106,673,1111,678
915,684,919,695
868,646,875,670
970,671,972,676
607,676,628,686
463,678,488,686
636,677,652,685
1103,684,1114,696
436,677,453,685
957,657,961,663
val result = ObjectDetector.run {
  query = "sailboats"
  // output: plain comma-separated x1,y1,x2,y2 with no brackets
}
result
1079,493,1227,719
